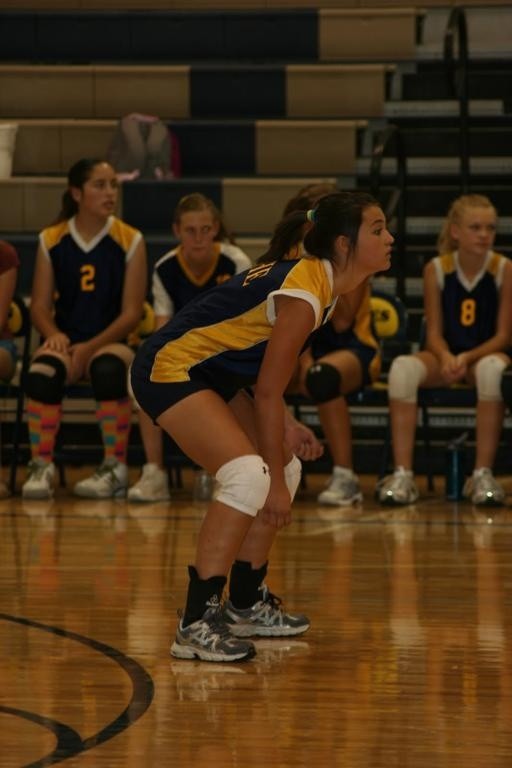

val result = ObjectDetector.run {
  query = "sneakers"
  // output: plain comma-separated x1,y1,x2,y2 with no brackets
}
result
316,472,364,507
218,589,309,639
127,469,172,503
74,465,130,500
377,474,420,505
171,600,258,663
462,472,505,509
22,460,59,500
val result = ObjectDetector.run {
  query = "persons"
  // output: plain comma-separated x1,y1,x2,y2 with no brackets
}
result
124,188,394,662
125,193,265,501
282,181,383,509
24,158,147,499
1,239,19,498
374,192,511,507
